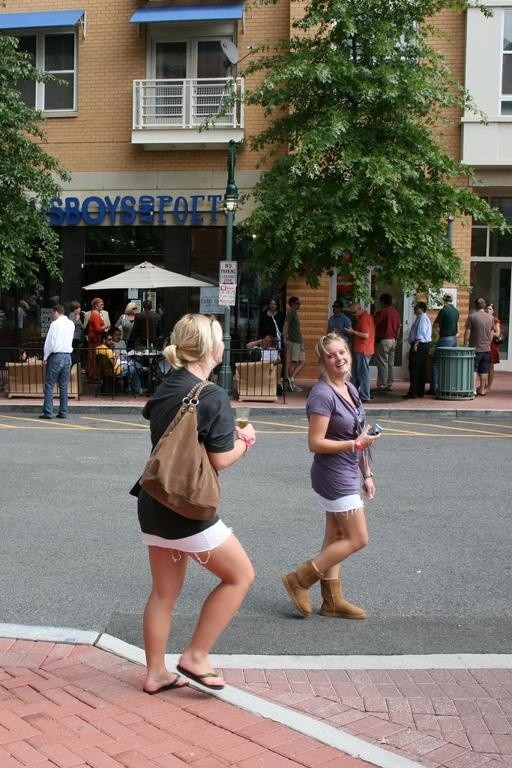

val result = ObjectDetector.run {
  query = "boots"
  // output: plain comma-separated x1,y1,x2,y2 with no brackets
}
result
319,576,367,619
282,558,324,616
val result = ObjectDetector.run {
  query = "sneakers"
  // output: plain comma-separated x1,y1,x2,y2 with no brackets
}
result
286,383,303,392
56,414,66,418
401,393,424,398
38,415,50,419
370,389,392,394
287,377,295,392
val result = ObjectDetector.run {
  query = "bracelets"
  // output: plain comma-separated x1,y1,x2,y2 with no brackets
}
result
362,472,374,480
236,433,251,456
350,438,363,453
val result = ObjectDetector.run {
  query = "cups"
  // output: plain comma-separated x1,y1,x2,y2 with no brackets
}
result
234,405,249,429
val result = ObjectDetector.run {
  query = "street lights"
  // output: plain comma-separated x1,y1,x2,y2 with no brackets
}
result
215,137,239,402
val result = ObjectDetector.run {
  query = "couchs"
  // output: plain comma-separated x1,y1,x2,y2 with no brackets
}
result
4,361,83,399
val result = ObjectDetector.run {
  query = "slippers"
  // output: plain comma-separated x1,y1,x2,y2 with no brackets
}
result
143,673,189,694
476,389,486,396
177,664,224,690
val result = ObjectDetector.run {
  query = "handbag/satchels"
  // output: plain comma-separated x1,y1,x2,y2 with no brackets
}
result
139,403,221,520
276,332,283,351
495,334,505,344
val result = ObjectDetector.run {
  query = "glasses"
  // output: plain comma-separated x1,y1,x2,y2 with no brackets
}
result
351,311,357,314
293,302,300,305
204,312,218,328
132,307,137,310
332,305,339,308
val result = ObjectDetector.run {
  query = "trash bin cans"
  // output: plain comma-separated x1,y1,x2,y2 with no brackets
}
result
437,347,476,401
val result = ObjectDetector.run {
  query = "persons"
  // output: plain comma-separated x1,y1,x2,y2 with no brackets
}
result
462,295,494,396
342,301,376,401
245,334,282,365
481,303,501,393
63,298,190,398
280,331,376,619
38,304,76,419
130,312,256,696
372,293,401,392
326,300,351,341
399,301,431,399
280,296,306,393
428,292,459,395
80,258,216,348
256,298,284,345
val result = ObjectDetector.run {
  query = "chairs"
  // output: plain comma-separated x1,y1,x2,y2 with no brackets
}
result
92,337,177,399
233,360,283,403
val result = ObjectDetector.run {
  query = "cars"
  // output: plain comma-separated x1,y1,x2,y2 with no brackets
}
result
220,298,256,335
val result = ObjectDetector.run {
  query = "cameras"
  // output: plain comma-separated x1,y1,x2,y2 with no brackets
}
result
367,424,384,435
